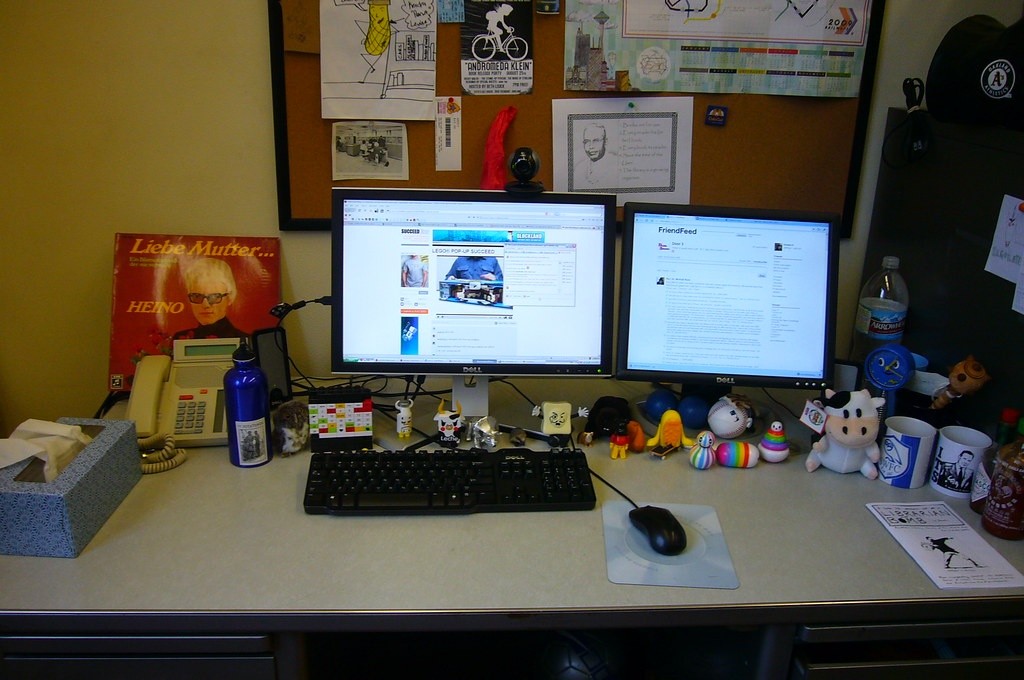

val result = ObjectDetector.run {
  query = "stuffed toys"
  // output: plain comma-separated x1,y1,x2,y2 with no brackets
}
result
805,389,885,481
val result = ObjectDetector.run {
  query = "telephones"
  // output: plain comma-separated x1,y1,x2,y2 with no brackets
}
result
126,338,249,450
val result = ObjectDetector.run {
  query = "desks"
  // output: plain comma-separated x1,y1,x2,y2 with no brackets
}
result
0,377,1023,680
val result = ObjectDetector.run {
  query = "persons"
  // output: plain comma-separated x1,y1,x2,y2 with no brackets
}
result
166,257,250,340
402,254,428,287
444,256,503,281
336,134,390,168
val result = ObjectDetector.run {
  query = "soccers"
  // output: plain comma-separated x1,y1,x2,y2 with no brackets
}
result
538,629,627,680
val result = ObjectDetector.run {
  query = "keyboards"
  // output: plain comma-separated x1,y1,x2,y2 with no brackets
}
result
303,447,596,516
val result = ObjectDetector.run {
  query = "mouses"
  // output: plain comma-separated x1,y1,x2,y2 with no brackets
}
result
629,505,687,556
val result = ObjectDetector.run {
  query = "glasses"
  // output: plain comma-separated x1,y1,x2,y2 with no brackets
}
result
188,293,229,304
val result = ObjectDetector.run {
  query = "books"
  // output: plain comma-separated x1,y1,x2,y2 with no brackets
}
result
864,501,1024,590
108,232,282,391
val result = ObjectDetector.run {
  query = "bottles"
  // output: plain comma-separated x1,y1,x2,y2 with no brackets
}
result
989,408,1020,461
223,341,274,468
850,255,909,378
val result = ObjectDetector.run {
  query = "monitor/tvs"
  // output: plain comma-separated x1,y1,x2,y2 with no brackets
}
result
615,201,843,450
331,187,617,451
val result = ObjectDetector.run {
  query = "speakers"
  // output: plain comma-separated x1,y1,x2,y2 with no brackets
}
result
251,327,292,411
820,358,862,399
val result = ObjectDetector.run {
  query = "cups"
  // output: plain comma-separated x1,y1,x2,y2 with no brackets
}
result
876,416,938,489
910,352,930,371
928,425,993,499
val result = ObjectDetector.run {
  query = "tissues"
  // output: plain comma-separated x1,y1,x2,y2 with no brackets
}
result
0,416,143,559
